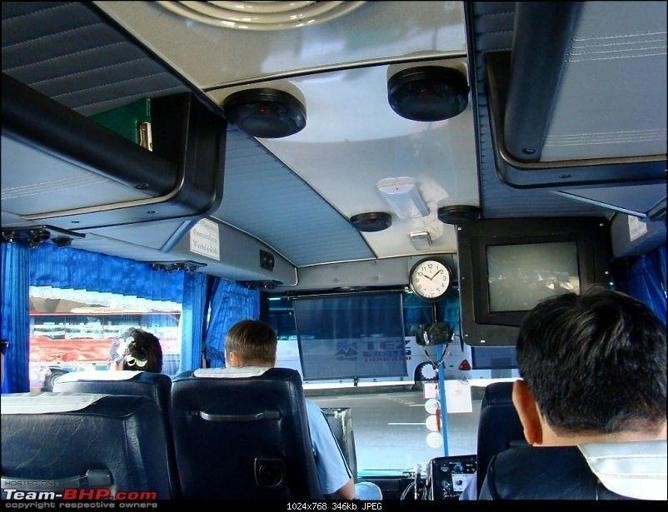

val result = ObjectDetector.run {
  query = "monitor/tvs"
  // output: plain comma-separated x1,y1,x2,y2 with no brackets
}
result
470,233,594,327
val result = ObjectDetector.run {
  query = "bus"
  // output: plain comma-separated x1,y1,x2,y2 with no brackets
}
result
29,308,183,394
266,289,520,393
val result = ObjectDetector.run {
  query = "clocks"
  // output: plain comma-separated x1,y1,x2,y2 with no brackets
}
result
408,256,455,325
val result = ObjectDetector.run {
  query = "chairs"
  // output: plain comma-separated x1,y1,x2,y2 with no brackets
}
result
167,365,325,500
1,391,178,499
51,369,177,499
478,439,667,500
476,380,527,499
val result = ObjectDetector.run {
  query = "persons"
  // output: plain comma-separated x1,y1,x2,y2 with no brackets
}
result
224,320,383,501
107,328,163,373
512,288,667,448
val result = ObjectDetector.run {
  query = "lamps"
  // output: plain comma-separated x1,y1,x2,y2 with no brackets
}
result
409,230,433,252
376,182,429,219
